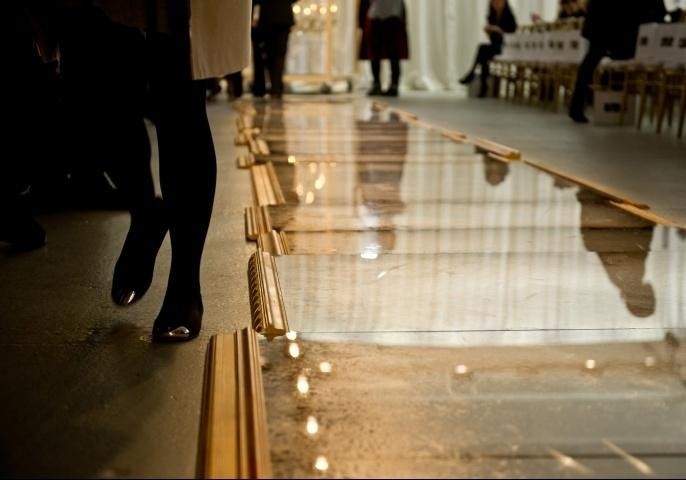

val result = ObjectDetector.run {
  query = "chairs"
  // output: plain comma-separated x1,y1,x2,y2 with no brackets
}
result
474,0,686,135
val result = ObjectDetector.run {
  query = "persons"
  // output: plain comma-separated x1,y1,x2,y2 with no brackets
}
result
576,184,656,322
473,146,513,189
250,0,298,99
458,0,519,99
1,1,132,254
208,72,243,98
355,104,412,253
247,98,301,245
565,1,670,125
529,1,586,104
353,0,412,99
92,1,251,341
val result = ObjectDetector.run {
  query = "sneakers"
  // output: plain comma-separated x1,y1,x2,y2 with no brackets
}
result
110,197,169,305
152,313,200,343
